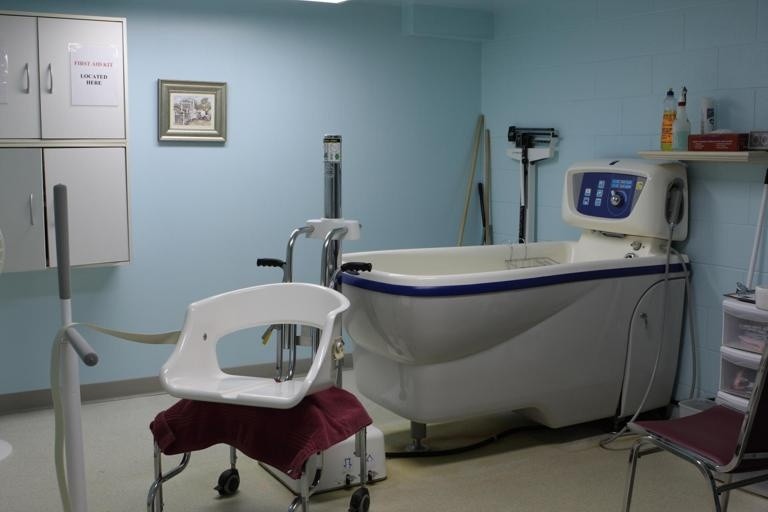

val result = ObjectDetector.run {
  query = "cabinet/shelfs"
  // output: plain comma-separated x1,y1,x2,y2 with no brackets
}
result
1,10,132,275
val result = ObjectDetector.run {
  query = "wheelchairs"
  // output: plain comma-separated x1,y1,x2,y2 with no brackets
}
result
147,217,373,512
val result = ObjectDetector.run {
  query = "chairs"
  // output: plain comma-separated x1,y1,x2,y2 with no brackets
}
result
619,316,767,510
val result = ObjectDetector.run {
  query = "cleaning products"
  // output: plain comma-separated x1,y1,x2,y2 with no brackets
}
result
703,97,714,131
671,85,690,151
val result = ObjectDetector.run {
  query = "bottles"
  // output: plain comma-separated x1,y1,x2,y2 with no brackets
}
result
700,96,714,135
660,89,677,150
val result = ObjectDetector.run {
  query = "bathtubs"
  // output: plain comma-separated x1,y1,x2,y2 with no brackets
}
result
342,238,688,429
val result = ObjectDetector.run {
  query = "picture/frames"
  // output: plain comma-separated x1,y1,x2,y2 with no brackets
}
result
157,80,229,145
